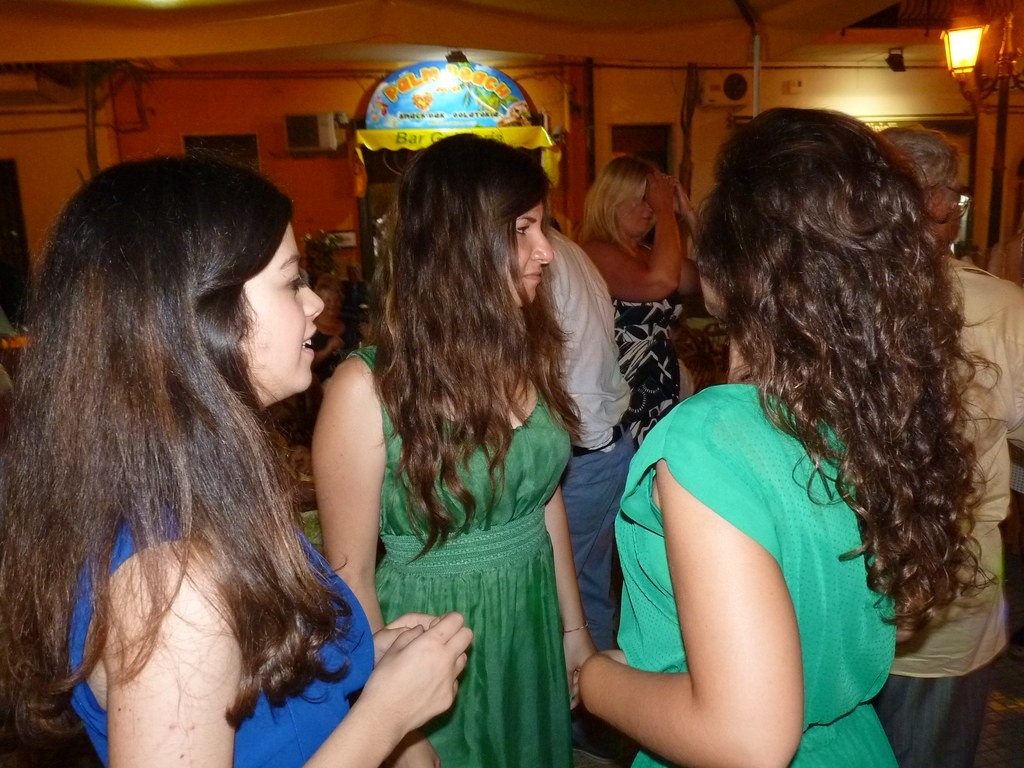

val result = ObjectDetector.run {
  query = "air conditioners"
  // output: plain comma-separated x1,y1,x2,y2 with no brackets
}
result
702,69,754,108
284,110,337,154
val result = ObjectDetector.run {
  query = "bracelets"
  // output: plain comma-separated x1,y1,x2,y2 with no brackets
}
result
559,622,590,632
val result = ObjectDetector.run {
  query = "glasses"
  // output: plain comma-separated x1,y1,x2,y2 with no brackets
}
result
932,182,972,217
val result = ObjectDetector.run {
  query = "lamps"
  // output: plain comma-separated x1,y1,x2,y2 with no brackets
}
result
446,50,469,64
885,54,906,73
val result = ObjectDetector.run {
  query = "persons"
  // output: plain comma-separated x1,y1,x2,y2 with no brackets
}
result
0,108,1024,768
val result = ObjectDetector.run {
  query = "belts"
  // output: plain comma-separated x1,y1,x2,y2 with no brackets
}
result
573,418,628,457
